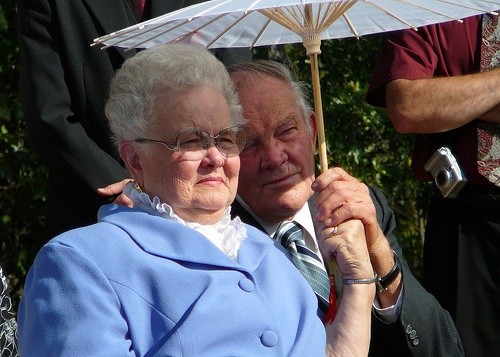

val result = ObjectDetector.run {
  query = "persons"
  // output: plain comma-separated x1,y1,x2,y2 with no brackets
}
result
17,42,377,357
226,59,466,357
367,10,500,357
18,0,251,244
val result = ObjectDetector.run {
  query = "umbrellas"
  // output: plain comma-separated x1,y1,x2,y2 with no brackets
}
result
89,0,500,174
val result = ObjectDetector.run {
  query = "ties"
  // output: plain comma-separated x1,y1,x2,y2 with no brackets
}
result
273,221,331,316
477,9,500,187
130,0,150,23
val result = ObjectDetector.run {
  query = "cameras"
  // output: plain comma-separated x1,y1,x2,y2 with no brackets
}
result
425,146,468,199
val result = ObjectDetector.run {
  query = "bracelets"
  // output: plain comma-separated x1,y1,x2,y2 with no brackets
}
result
343,272,379,285
376,250,402,293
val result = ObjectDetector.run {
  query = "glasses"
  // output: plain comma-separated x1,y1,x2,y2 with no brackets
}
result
136,126,246,161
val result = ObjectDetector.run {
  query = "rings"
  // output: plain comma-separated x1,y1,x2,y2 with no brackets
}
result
334,226,338,236
